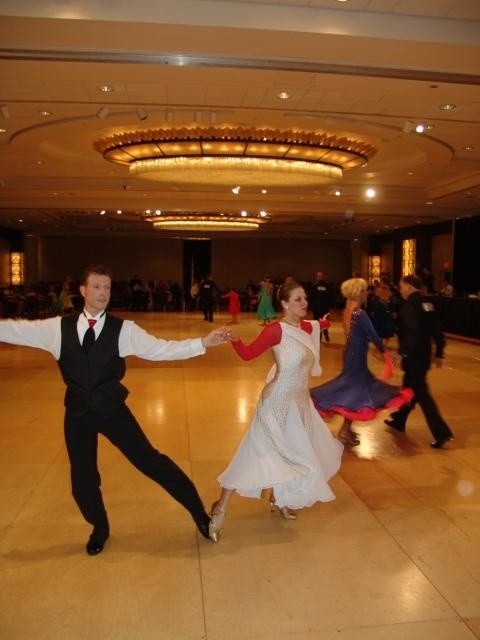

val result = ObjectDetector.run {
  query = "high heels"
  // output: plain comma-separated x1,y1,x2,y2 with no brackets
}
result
207,498,226,543
269,495,298,520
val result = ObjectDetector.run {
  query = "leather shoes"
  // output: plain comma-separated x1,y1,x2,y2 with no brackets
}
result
338,429,361,447
430,433,454,449
193,511,212,541
85,527,110,557
382,417,407,432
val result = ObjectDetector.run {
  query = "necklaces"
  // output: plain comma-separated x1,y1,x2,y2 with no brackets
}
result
283,315,300,328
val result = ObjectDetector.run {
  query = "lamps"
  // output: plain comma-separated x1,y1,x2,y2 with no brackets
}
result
93,127,379,185
140,207,273,232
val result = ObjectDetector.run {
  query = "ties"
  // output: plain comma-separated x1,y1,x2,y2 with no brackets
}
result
80,318,97,347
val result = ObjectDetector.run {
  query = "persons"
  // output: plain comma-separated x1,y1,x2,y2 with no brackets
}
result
308,277,415,446
208,275,344,544
382,272,454,448
0,261,229,556
